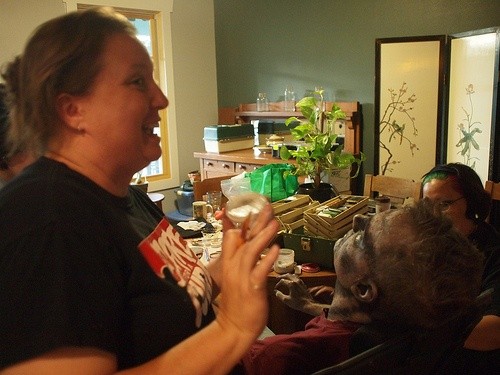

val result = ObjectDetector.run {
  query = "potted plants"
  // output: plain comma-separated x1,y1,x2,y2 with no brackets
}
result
281,87,370,205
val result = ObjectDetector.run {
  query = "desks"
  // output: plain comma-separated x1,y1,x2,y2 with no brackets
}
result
182,233,338,336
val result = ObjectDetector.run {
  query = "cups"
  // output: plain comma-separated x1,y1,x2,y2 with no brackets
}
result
375,196,391,214
192,201,213,221
206,191,222,213
273,248,295,275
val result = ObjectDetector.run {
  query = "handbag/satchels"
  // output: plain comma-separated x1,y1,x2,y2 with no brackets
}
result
244,163,299,202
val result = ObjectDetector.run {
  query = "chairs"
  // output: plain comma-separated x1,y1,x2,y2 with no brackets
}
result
193,175,241,205
364,174,423,209
312,287,497,375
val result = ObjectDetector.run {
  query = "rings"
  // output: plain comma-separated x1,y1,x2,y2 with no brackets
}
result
250,282,265,291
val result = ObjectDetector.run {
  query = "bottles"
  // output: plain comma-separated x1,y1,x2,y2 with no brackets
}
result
285,88,295,112
256,92,269,112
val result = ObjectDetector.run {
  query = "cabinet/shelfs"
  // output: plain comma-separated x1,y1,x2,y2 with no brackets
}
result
193,100,361,185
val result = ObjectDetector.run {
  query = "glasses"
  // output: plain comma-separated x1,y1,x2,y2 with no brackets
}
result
425,197,463,211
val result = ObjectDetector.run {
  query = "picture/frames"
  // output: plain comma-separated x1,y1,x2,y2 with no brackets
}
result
439,26,500,188
372,34,448,199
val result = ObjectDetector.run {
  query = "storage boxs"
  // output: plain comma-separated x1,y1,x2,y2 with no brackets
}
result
258,121,300,147
203,124,255,154
268,193,370,272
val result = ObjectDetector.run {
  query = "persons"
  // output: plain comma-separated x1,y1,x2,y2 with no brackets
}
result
310,161,500,375
238,198,490,375
0,5,280,375
